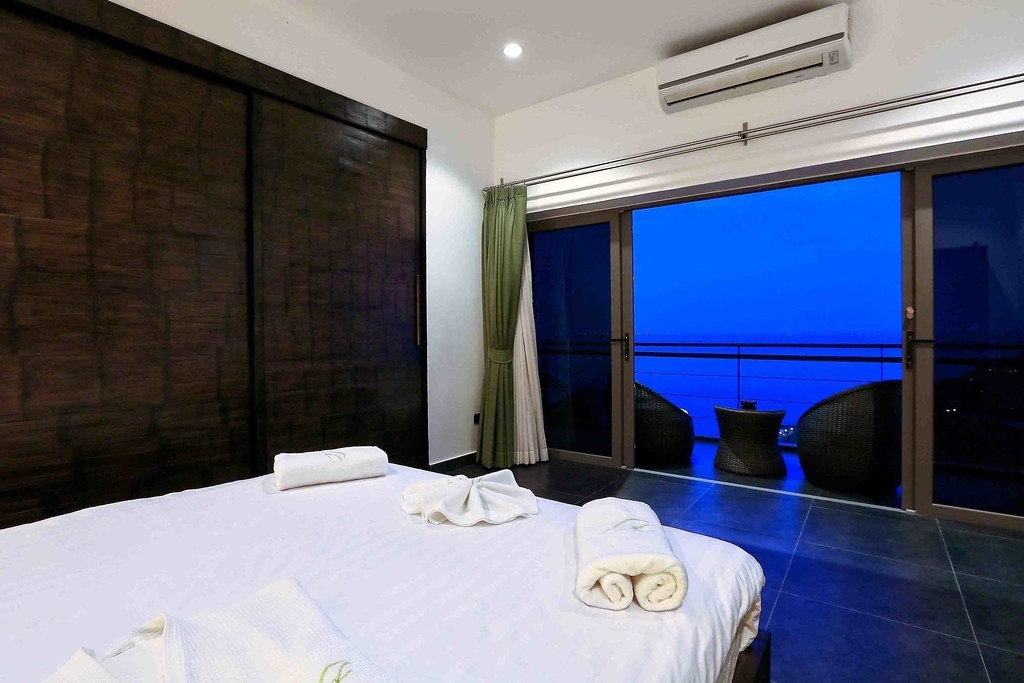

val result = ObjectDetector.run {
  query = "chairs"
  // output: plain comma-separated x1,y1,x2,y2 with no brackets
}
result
634,382,695,470
796,379,903,498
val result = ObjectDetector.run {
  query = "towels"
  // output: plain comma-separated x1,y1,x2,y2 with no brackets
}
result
273,445,388,490
575,495,688,612
400,468,540,527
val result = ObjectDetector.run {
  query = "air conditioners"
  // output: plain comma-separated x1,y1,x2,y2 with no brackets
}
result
656,2,852,115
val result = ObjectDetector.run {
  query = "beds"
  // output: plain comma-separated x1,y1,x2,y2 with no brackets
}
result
0,446,765,683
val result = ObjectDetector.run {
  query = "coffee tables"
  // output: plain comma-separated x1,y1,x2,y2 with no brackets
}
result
713,404,786,480
